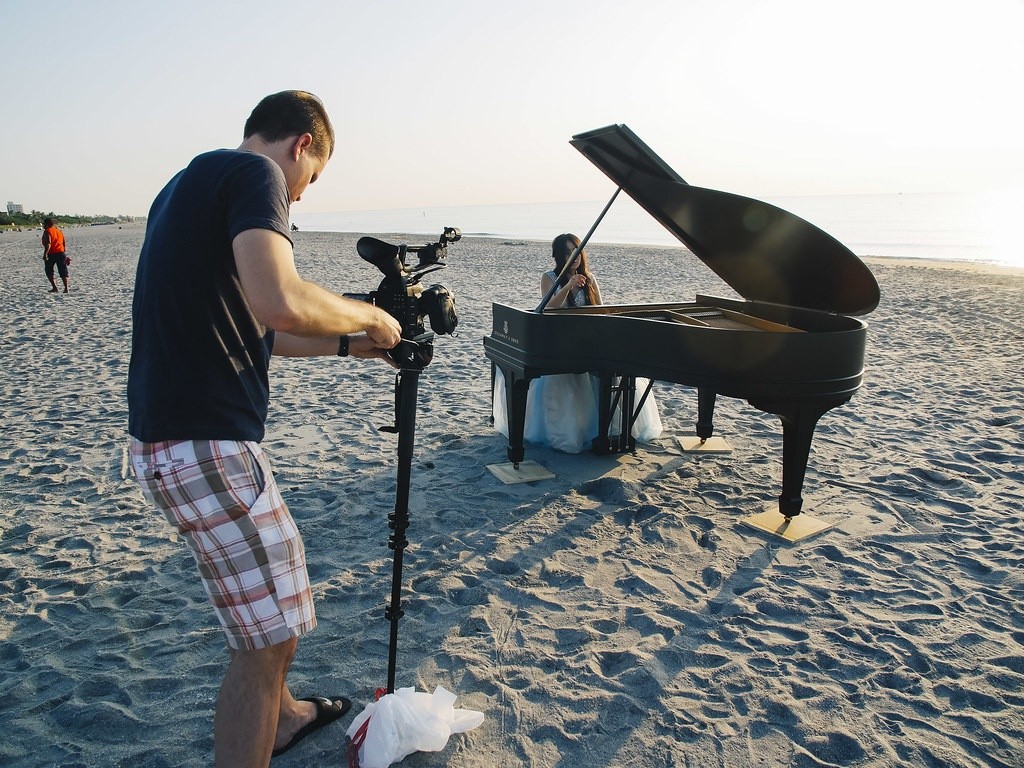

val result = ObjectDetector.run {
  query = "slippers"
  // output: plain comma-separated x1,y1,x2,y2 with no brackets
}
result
271,696,351,758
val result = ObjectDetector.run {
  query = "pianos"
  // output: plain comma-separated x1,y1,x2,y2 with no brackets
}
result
480,121,882,525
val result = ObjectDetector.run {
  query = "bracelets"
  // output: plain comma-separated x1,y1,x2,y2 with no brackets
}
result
338,334,349,357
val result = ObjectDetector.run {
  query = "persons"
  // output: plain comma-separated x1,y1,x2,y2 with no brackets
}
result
126,88,402,768
42,218,69,293
491,234,662,451
